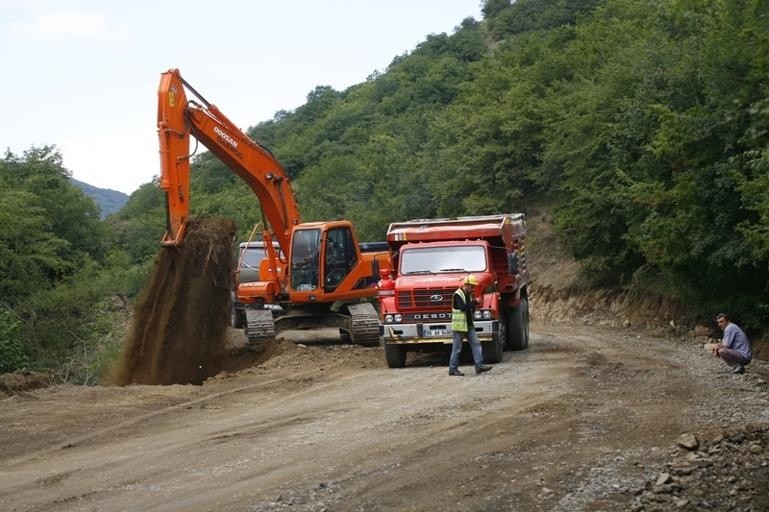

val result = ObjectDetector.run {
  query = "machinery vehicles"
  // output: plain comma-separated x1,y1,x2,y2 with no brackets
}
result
157,69,390,348
231,240,283,328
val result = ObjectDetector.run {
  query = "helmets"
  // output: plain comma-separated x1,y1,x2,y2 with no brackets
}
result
464,274,479,286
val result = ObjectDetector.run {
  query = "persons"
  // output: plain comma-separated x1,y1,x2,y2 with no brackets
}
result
448,276,492,376
716,313,752,374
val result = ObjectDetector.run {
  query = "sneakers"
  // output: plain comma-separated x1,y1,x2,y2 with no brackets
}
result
730,366,746,374
475,365,492,374
449,369,465,376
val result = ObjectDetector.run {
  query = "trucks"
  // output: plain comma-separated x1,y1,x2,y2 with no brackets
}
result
380,212,534,369
